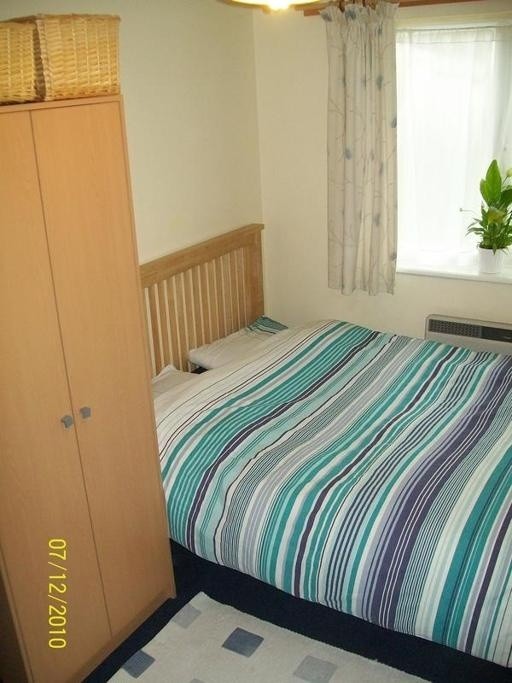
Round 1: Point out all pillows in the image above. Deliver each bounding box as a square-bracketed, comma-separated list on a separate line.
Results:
[151, 313, 290, 400]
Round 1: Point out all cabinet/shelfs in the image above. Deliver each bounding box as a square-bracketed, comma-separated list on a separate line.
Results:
[0, 93, 179, 683]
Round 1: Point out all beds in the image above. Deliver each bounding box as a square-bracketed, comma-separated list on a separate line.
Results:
[139, 222, 512, 672]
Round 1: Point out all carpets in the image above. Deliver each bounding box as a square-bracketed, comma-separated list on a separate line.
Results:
[109, 590, 433, 683]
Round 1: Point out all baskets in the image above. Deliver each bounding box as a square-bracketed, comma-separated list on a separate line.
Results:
[0, 15, 121, 103]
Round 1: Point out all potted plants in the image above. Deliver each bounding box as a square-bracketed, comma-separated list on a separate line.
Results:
[463, 159, 512, 274]
